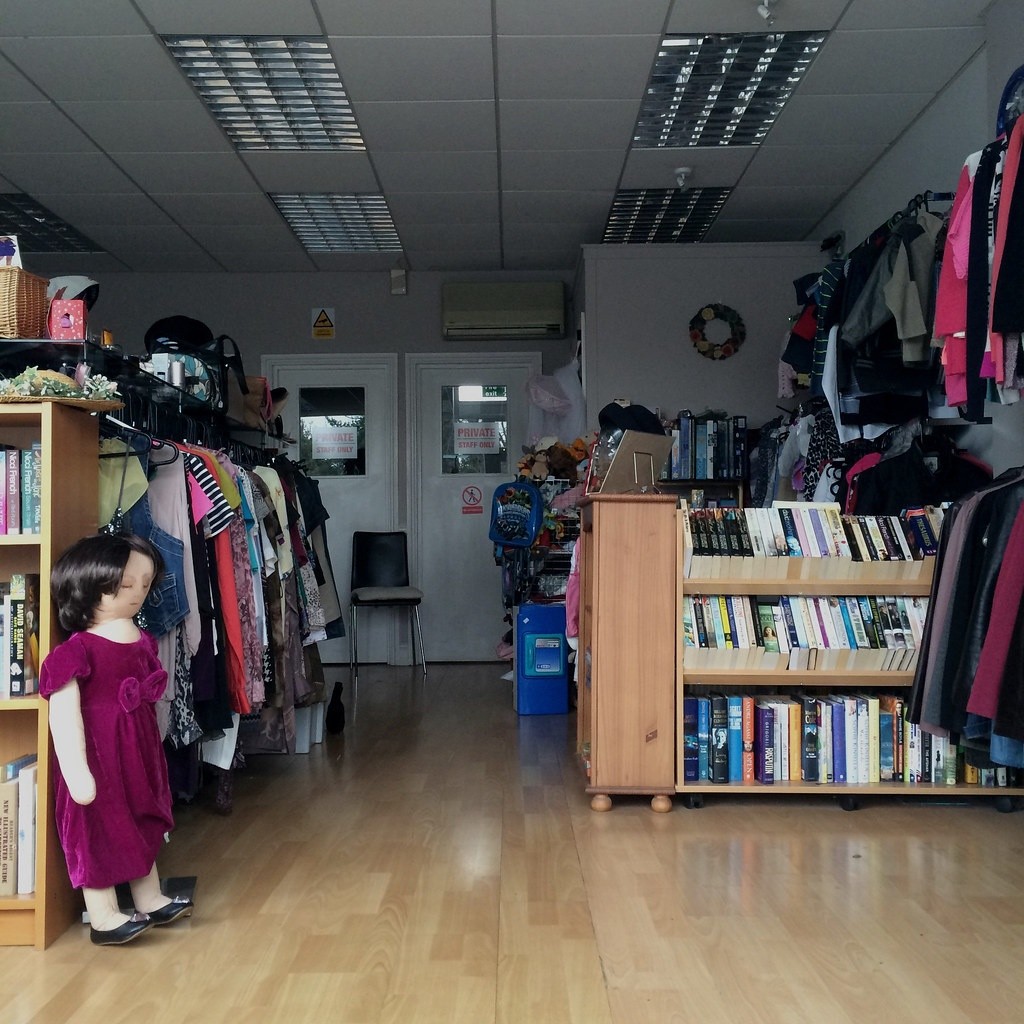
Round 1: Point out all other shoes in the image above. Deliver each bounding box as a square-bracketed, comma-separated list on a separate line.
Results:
[134, 896, 195, 925]
[90, 912, 154, 945]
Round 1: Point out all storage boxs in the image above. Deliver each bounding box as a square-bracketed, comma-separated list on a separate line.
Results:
[295, 702, 325, 754]
[45, 285, 88, 340]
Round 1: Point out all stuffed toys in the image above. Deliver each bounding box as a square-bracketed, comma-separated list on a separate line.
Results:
[515, 430, 598, 484]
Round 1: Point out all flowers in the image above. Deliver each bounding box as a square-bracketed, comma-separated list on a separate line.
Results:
[0, 363, 117, 399]
[688, 303, 748, 360]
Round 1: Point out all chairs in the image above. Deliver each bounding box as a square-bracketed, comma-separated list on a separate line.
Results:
[346, 529, 429, 679]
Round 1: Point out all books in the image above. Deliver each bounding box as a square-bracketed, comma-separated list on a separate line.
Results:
[680, 489, 953, 581]
[0, 574, 40, 700]
[683, 594, 930, 671]
[0, 752, 38, 898]
[659, 415, 748, 481]
[684, 694, 1024, 787]
[0, 438, 41, 535]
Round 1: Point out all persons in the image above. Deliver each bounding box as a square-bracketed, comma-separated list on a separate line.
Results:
[40, 533, 194, 947]
[762, 626, 777, 643]
[713, 729, 726, 749]
[878, 549, 889, 561]
[879, 606, 891, 631]
[786, 536, 802, 557]
[830, 597, 839, 608]
[896, 702, 903, 715]
[743, 741, 752, 752]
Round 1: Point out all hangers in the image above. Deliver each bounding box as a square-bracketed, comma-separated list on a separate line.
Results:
[98, 387, 268, 470]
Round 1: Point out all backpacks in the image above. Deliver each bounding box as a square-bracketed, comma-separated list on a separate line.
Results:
[489, 475, 543, 546]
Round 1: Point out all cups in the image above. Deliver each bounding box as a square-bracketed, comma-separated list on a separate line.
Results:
[170, 361, 185, 388]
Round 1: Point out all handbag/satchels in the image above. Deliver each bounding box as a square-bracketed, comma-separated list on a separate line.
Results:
[226, 374, 272, 432]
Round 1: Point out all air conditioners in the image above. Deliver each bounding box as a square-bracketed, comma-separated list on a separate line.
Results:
[440, 282, 566, 341]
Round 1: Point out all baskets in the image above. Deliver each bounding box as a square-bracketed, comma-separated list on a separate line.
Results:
[0, 265, 50, 340]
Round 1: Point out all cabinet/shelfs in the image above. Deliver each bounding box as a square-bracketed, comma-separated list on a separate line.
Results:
[576, 494, 677, 811]
[679, 507, 1023, 811]
[0, 402, 101, 951]
[656, 476, 746, 506]
[525, 474, 580, 603]
